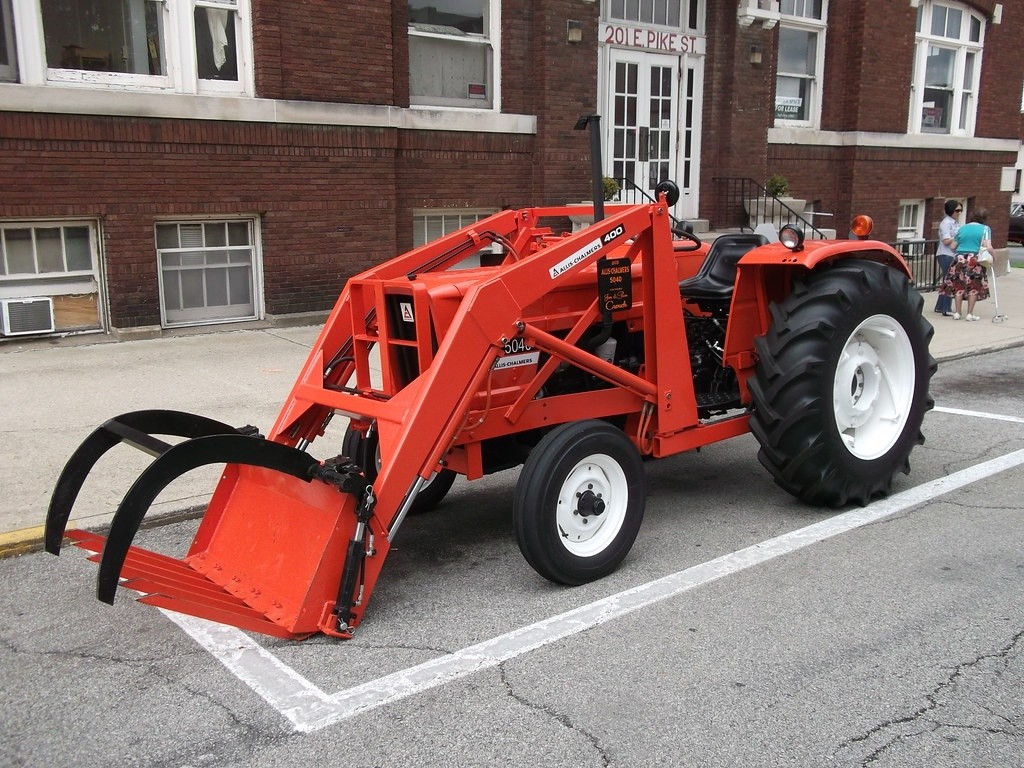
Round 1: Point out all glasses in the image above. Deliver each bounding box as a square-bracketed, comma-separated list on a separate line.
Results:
[955, 209, 963, 213]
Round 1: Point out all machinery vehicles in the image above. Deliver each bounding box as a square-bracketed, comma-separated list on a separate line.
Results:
[44, 114, 940, 643]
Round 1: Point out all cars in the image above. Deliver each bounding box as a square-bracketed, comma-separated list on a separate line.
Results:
[1008, 202, 1024, 247]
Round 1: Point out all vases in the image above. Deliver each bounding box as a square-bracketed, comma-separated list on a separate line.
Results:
[743, 173, 807, 231]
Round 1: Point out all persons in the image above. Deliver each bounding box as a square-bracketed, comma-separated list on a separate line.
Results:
[934, 200, 964, 317]
[937, 208, 996, 322]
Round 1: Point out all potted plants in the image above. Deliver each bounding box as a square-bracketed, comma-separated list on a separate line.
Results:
[565, 177, 624, 232]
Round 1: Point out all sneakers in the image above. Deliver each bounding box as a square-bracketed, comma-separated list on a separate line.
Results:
[966, 314, 980, 321]
[943, 311, 954, 316]
[953, 312, 963, 319]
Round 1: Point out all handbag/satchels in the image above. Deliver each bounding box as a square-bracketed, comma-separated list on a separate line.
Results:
[977, 246, 994, 267]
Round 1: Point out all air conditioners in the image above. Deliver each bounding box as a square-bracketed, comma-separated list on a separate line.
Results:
[899, 238, 926, 259]
[0, 297, 55, 336]
